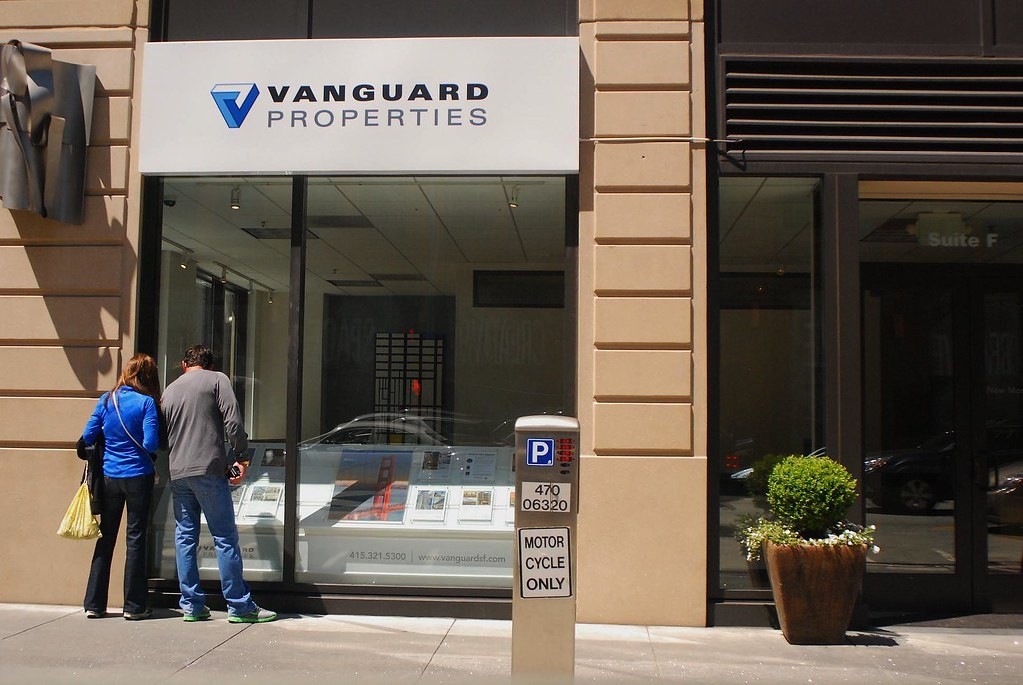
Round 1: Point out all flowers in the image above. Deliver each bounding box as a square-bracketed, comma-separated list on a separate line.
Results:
[739, 453, 881, 561]
[732, 456, 787, 556]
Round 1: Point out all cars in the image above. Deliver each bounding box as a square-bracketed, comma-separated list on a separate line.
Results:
[729, 441, 1023, 530]
[298, 404, 565, 446]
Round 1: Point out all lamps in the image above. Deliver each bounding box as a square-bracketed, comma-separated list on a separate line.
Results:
[248, 280, 253, 294]
[507, 186, 521, 208]
[220, 269, 228, 283]
[231, 188, 242, 210]
[267, 291, 272, 303]
[181, 253, 192, 270]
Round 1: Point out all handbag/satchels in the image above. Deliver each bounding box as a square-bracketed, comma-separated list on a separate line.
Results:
[56, 461, 103, 541]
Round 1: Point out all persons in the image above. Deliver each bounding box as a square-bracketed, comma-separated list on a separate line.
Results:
[159, 345, 277, 623]
[82, 353, 160, 621]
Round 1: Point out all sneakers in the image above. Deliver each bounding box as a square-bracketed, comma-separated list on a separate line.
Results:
[228, 605, 278, 623]
[183, 605, 211, 621]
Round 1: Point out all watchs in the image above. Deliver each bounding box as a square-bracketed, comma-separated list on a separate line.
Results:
[241, 461, 249, 467]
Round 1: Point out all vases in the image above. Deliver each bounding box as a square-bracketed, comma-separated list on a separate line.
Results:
[745, 552, 772, 591]
[761, 539, 867, 645]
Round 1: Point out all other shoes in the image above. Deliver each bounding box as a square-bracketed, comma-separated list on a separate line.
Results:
[123, 607, 153, 620]
[85, 609, 107, 618]
